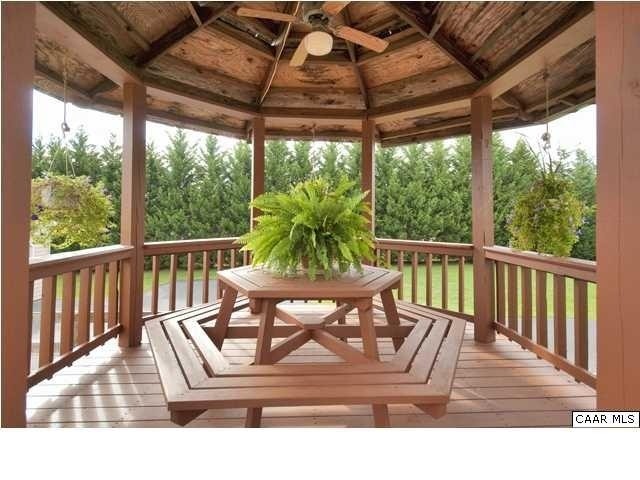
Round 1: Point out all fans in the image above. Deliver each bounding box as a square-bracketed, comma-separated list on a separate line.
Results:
[236, 1, 390, 67]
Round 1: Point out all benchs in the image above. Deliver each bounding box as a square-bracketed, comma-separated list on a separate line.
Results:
[143, 296, 467, 427]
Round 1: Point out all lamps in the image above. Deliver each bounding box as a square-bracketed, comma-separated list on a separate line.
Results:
[302, 1, 334, 56]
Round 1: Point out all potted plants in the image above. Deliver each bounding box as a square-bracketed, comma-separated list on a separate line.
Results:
[31, 174, 119, 251]
[233, 174, 382, 280]
[503, 157, 587, 259]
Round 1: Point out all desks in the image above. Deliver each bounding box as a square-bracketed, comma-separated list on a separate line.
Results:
[200, 258, 419, 428]
